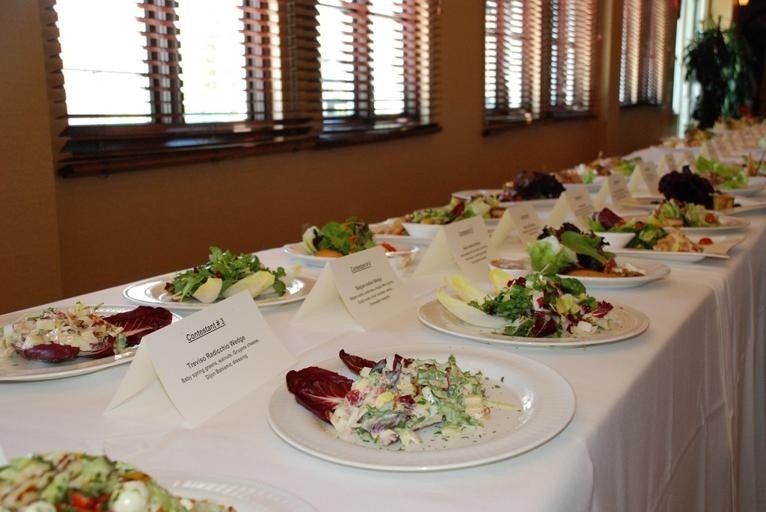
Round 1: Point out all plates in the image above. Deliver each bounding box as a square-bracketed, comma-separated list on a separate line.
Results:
[618, 214, 751, 232]
[711, 179, 763, 197]
[624, 195, 765, 216]
[489, 255, 669, 295]
[558, 176, 605, 194]
[151, 470, 315, 512]
[453, 187, 559, 208]
[0, 302, 184, 384]
[265, 341, 579, 474]
[416, 293, 651, 348]
[280, 234, 421, 267]
[540, 231, 748, 267]
[121, 271, 317, 311]
[368, 216, 479, 244]
